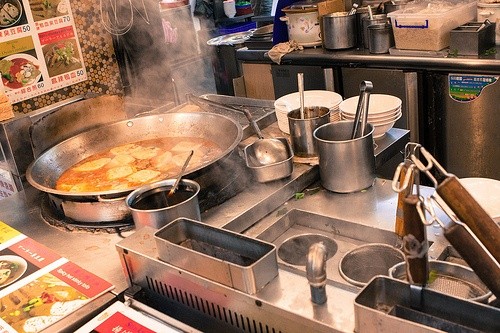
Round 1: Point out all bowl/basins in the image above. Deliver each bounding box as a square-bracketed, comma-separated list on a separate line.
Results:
[274, 90, 343, 134]
[0, 0, 22, 27]
[125, 179, 201, 233]
[289, 107, 330, 158]
[435, 177, 500, 222]
[313, 120, 374, 193]
[340, 95, 403, 138]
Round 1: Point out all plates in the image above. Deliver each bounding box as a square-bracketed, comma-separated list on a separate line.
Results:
[0, 54, 42, 89]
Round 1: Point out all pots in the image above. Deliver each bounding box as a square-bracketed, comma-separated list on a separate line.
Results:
[26, 112, 242, 203]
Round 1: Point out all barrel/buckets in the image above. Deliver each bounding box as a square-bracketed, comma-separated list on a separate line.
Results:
[321, 12, 360, 49]
[207, 32, 256, 97]
[363, 14, 391, 53]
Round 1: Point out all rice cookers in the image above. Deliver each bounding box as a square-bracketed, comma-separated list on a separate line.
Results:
[280, 4, 322, 49]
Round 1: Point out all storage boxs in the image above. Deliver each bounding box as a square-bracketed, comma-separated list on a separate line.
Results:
[386, 0, 479, 51]
